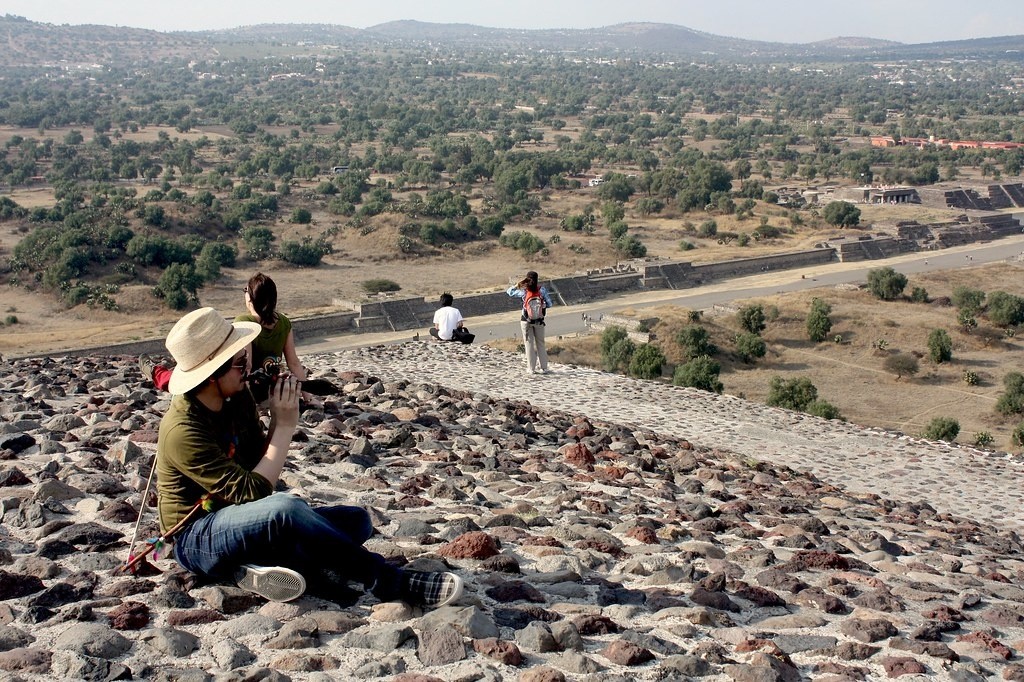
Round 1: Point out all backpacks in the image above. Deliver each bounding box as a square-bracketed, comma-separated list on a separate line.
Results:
[523, 285, 543, 320]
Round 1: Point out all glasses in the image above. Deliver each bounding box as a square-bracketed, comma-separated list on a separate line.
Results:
[219, 348, 247, 375]
[243, 287, 249, 294]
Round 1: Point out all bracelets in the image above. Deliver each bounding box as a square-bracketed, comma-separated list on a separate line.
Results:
[304, 398, 312, 403]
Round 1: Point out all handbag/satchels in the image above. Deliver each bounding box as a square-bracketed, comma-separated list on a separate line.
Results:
[452, 327, 475, 344]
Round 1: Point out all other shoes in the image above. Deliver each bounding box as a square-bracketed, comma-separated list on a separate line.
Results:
[138, 353, 158, 382]
[540, 368, 548, 374]
[527, 369, 535, 375]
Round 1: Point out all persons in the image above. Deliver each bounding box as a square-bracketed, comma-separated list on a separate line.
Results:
[507, 271, 552, 373]
[138, 272, 325, 409]
[429, 294, 463, 340]
[157, 307, 463, 609]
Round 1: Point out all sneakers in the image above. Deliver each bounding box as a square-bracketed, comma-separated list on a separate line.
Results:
[234, 564, 306, 603]
[381, 566, 463, 610]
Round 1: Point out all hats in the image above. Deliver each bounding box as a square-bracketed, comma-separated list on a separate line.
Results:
[527, 271, 538, 279]
[167, 307, 262, 395]
